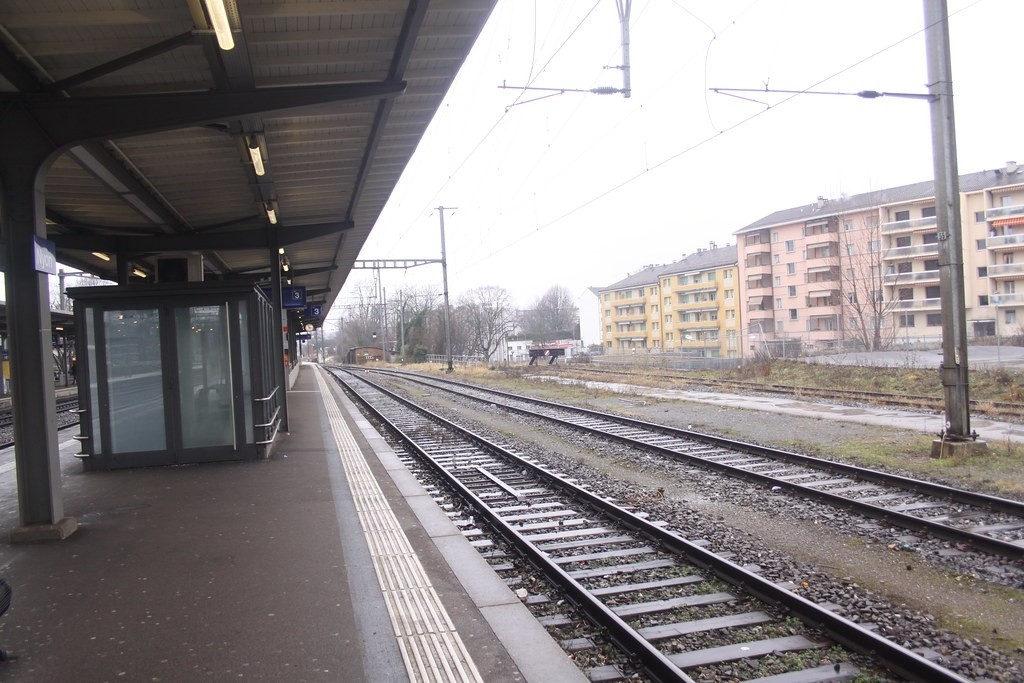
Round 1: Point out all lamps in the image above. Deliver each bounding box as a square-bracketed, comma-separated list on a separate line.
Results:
[265, 199, 278, 224]
[204, 0, 235, 51]
[248, 135, 265, 177]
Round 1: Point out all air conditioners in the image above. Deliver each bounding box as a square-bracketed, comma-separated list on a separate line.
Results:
[155, 255, 204, 282]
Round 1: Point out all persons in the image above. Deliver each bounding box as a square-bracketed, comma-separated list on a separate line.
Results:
[71, 358, 77, 385]
